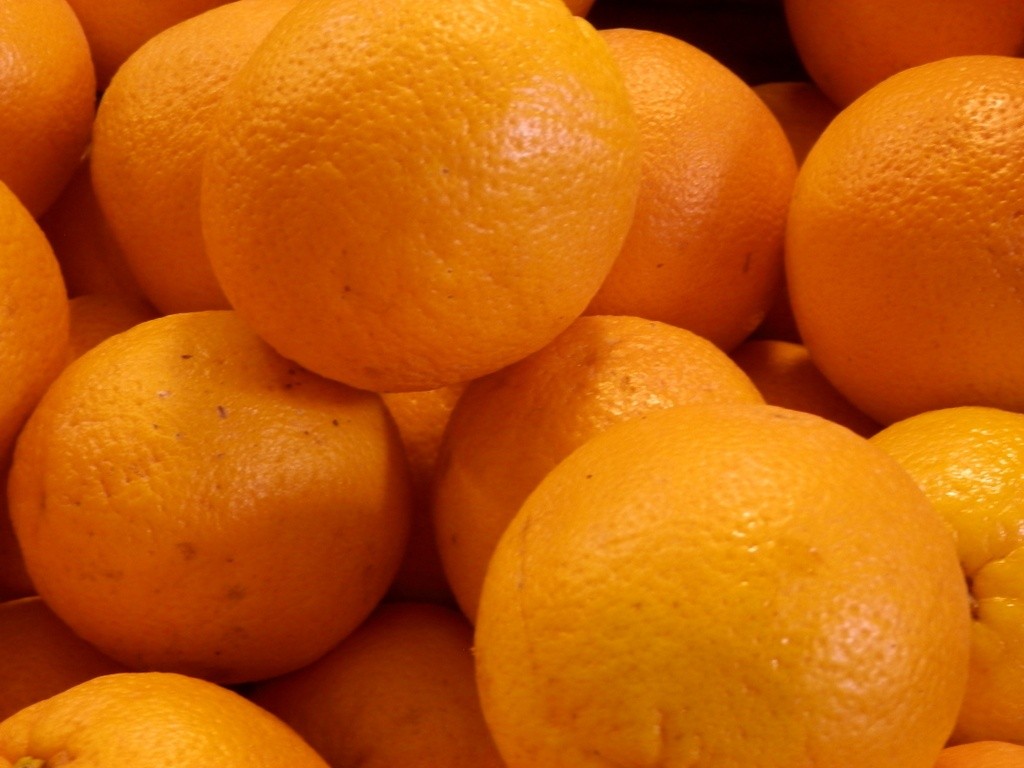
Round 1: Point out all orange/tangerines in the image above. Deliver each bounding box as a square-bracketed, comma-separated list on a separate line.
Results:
[0, 0, 1024, 768]
